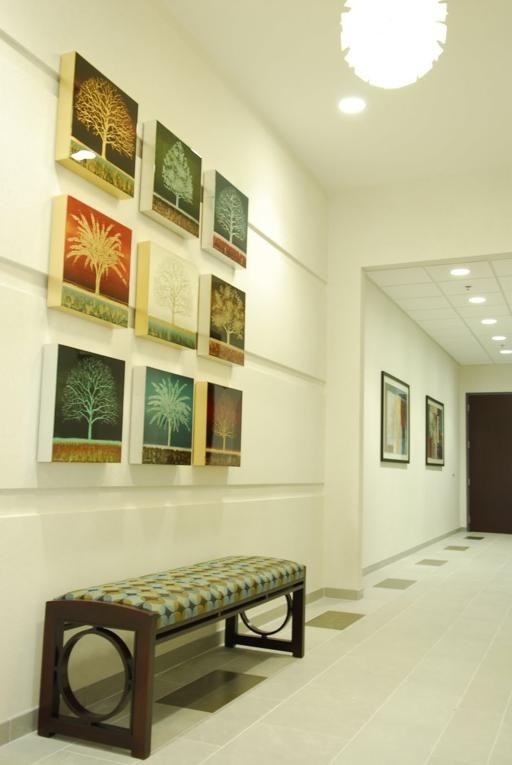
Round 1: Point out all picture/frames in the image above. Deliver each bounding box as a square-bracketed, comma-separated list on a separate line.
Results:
[380, 370, 410, 463]
[425, 395, 445, 466]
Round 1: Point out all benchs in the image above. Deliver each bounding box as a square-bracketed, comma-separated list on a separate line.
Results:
[36, 557, 308, 760]
